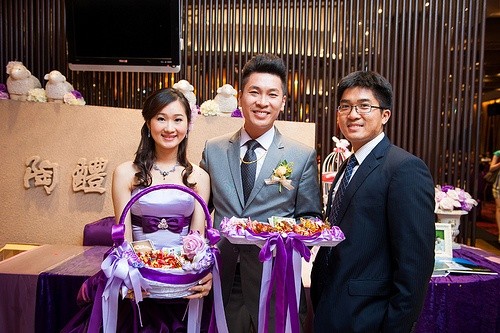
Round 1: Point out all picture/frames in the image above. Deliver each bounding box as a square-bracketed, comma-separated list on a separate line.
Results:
[435, 223, 452, 259]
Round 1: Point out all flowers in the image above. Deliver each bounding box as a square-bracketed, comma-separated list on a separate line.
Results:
[200, 99, 219, 116]
[265, 161, 295, 194]
[64, 90, 86, 106]
[180, 232, 206, 260]
[433, 184, 478, 212]
[27, 87, 47, 102]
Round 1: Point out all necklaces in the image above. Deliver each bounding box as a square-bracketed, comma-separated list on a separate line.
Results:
[153, 162, 179, 180]
[240, 152, 266, 164]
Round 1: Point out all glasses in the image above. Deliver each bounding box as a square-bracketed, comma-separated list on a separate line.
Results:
[338, 103, 387, 114]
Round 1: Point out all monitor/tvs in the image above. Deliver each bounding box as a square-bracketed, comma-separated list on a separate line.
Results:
[64, 0, 182, 73]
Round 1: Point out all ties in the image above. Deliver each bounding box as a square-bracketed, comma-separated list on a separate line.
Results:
[241, 140, 261, 206]
[328, 153, 359, 227]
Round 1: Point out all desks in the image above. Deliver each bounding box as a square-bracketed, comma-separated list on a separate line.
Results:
[0, 244, 500, 333]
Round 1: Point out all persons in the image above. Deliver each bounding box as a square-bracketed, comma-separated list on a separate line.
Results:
[64, 88, 212, 333]
[491, 151, 500, 242]
[200, 54, 322, 333]
[310, 71, 435, 333]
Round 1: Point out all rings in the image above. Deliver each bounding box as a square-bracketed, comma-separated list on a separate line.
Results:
[128, 290, 131, 293]
[129, 294, 133, 299]
[200, 293, 202, 299]
[201, 285, 204, 292]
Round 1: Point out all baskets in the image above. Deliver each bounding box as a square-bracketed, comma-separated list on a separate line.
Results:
[119, 184, 213, 299]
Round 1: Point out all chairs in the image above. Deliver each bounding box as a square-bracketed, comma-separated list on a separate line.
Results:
[83, 216, 116, 247]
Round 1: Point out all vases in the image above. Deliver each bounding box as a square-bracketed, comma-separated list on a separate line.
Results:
[436, 210, 468, 241]
